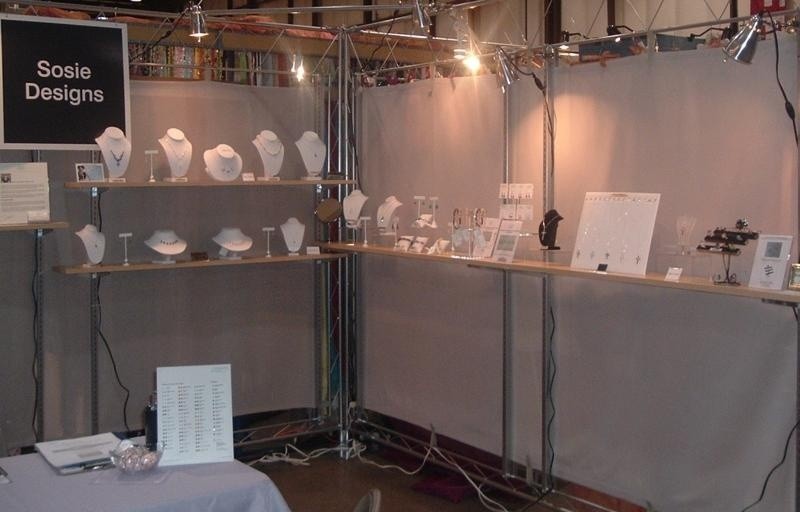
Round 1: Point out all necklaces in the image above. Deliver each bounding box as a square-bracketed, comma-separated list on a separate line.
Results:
[380, 199, 398, 223]
[575, 193, 657, 265]
[87, 134, 323, 250]
[541, 213, 561, 241]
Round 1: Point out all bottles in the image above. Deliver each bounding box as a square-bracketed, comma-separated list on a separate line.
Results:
[142, 391, 157, 451]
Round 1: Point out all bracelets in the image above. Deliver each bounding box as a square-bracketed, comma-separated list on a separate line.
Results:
[453, 208, 460, 230]
[397, 237, 411, 242]
[473, 207, 484, 228]
[411, 239, 424, 245]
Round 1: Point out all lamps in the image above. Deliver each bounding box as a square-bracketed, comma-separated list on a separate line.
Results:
[556, 24, 635, 51]
[719, 6, 799, 147]
[409, 0, 532, 95]
[187, 2, 209, 44]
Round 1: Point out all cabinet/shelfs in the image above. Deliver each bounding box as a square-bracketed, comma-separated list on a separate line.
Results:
[52, 179, 356, 462]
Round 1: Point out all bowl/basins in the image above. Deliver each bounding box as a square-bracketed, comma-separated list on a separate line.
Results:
[109, 446, 163, 474]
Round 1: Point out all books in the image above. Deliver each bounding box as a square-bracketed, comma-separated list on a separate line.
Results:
[33, 429, 133, 475]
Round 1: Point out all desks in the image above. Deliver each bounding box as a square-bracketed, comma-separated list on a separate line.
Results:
[0, 436, 272, 510]
